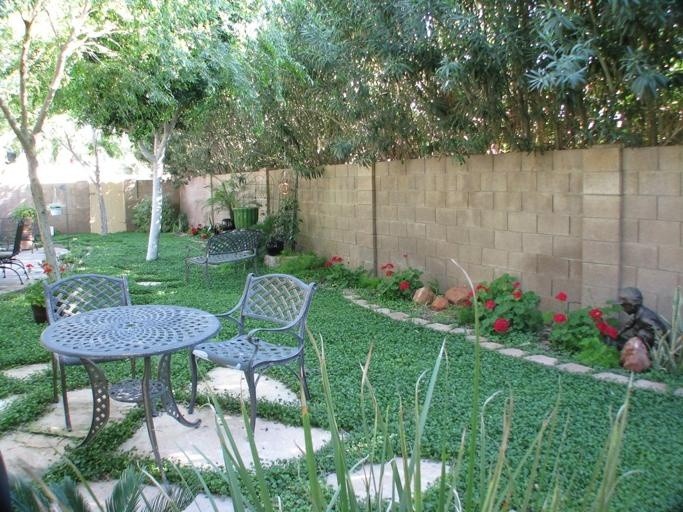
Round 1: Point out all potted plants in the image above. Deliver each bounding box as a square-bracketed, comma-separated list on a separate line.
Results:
[9, 203, 37, 250]
[253, 214, 284, 256]
[48, 202, 65, 216]
[26, 280, 45, 323]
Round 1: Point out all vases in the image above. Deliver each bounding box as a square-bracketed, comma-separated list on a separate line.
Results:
[222, 218, 233, 231]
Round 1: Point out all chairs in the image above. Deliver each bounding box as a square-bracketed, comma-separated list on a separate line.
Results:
[0, 219, 29, 285]
[43, 273, 159, 431]
[190, 272, 319, 439]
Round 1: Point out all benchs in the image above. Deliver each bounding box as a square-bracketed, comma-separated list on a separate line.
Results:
[186, 231, 260, 288]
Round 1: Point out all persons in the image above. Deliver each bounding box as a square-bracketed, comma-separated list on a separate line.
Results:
[612, 286, 670, 357]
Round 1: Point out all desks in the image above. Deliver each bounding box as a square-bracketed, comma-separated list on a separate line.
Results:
[39, 303, 221, 496]
